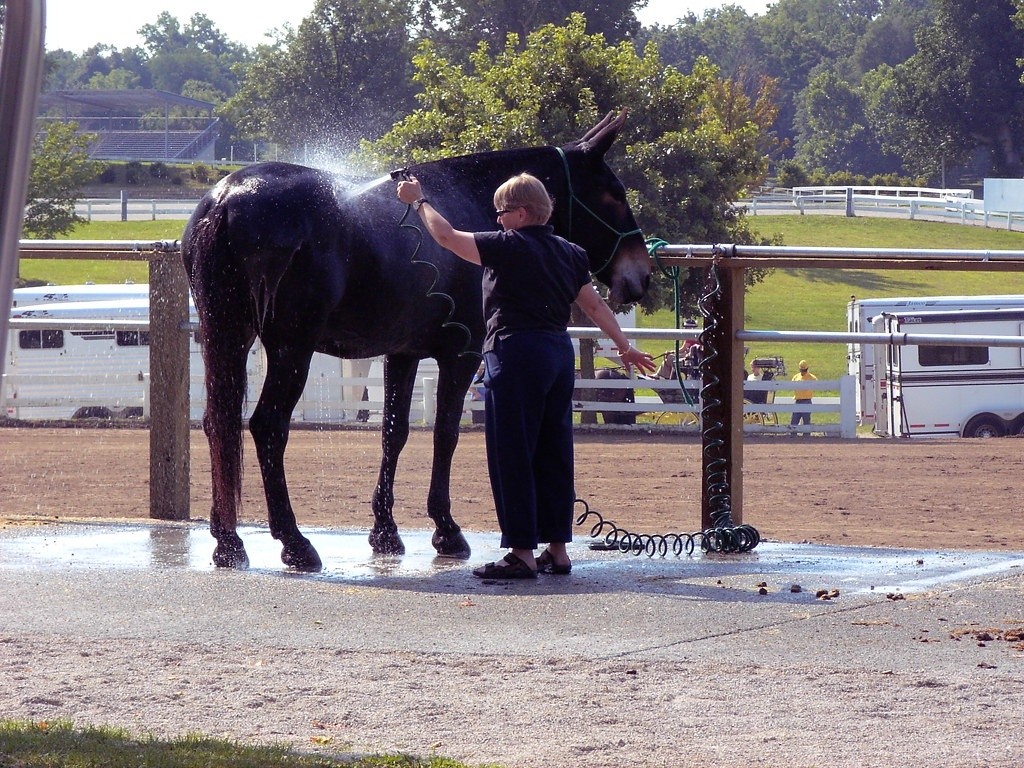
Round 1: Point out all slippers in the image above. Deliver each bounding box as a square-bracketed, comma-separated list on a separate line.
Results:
[473, 553, 538, 579]
[534, 549, 572, 574]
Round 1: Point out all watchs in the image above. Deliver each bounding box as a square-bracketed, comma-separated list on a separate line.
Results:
[413, 198, 429, 212]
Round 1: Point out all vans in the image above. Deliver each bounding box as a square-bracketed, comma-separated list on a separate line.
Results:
[0, 281, 343, 421]
[848, 294, 1024, 434]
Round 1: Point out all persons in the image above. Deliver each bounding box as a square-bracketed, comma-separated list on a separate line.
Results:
[646, 319, 703, 380]
[791, 359, 817, 436]
[747, 359, 763, 381]
[397, 172, 657, 579]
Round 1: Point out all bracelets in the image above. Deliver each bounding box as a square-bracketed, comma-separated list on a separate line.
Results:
[617, 343, 632, 357]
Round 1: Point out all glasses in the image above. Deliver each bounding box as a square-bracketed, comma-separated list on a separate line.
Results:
[496, 208, 516, 217]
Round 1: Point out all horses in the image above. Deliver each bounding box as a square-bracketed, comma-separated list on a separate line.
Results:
[182, 105, 652, 569]
